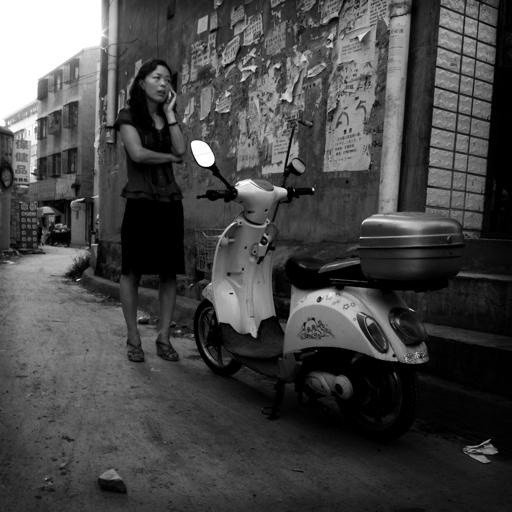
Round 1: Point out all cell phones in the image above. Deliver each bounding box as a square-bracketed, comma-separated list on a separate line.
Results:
[165, 90, 172, 103]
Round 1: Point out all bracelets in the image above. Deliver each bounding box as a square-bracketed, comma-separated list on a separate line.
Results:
[168, 122, 177, 127]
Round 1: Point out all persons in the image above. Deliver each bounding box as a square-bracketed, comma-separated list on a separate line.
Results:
[114, 58, 188, 362]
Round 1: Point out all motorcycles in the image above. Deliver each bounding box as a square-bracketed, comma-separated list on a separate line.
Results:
[189, 138, 465, 443]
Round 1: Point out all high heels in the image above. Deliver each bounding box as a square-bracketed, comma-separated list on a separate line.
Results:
[156, 342, 178, 361]
[127, 340, 144, 362]
